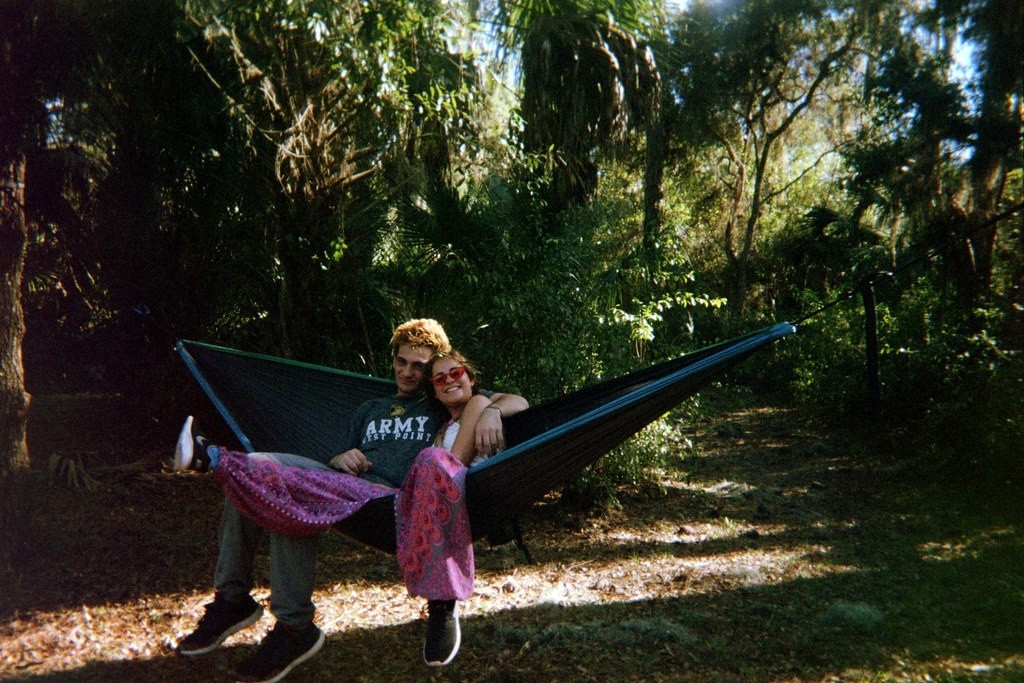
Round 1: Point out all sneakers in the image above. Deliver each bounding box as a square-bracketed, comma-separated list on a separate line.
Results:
[179, 592, 263, 655]
[422, 599, 462, 666]
[175, 415, 212, 474]
[235, 620, 325, 683]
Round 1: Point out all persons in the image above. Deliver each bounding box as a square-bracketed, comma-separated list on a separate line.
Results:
[180, 319, 528, 683]
[175, 351, 504, 665]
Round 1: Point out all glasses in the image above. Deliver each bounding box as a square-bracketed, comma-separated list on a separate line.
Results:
[430, 366, 465, 385]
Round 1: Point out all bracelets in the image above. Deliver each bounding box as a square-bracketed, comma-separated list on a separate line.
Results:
[487, 406, 502, 416]
